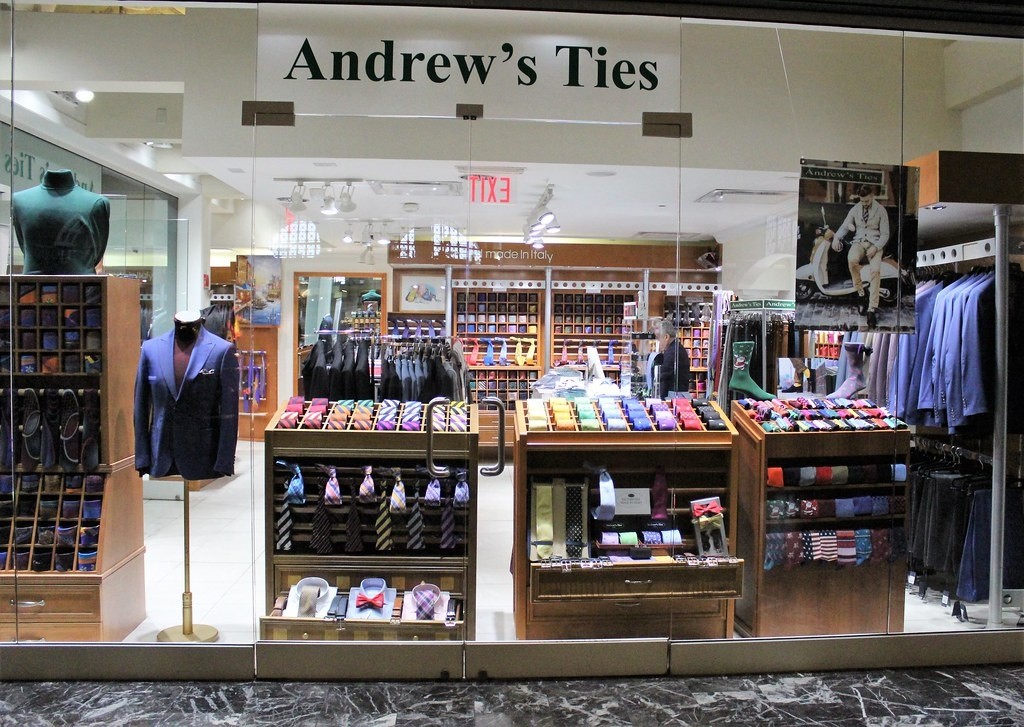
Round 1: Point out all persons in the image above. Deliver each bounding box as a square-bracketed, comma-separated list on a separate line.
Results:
[12, 169, 110, 275]
[368, 304, 376, 319]
[655, 321, 691, 402]
[832, 184, 889, 328]
[362, 290, 381, 310]
[133, 311, 239, 479]
[318, 314, 333, 346]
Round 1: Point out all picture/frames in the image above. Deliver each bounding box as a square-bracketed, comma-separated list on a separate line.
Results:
[398, 275, 447, 312]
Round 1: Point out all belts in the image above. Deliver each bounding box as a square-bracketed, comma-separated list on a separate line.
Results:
[727, 313, 797, 400]
[271, 596, 288, 617]
[707, 289, 739, 393]
[541, 556, 614, 574]
[444, 599, 457, 629]
[672, 552, 740, 567]
[390, 598, 403, 625]
[324, 596, 349, 631]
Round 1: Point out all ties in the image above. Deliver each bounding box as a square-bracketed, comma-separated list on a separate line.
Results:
[274, 460, 470, 554]
[238, 349, 243, 391]
[526, 397, 727, 431]
[251, 366, 261, 412]
[260, 350, 266, 398]
[665, 300, 712, 327]
[389, 318, 446, 340]
[0, 388, 100, 473]
[416, 588, 436, 620]
[689, 372, 706, 398]
[601, 531, 639, 545]
[277, 396, 468, 432]
[453, 337, 536, 366]
[677, 329, 708, 368]
[590, 464, 616, 521]
[641, 529, 682, 544]
[244, 365, 248, 411]
[458, 292, 537, 332]
[0, 474, 105, 572]
[248, 350, 254, 395]
[555, 295, 634, 334]
[553, 339, 619, 368]
[536, 485, 554, 561]
[608, 372, 616, 381]
[469, 370, 538, 410]
[566, 485, 582, 559]
[0, 285, 101, 373]
[651, 464, 668, 520]
[297, 585, 321, 618]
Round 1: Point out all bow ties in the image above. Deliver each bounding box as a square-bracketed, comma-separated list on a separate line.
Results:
[355, 591, 384, 608]
[692, 499, 724, 517]
[691, 513, 724, 530]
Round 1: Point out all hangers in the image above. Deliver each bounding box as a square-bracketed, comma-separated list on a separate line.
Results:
[346, 334, 460, 364]
[724, 311, 800, 328]
[910, 428, 1024, 488]
[914, 255, 993, 286]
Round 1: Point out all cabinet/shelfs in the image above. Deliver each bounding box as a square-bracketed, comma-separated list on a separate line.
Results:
[541, 292, 635, 385]
[454, 291, 541, 408]
[662, 298, 715, 401]
[511, 401, 745, 639]
[0, 274, 149, 643]
[265, 398, 479, 641]
[350, 311, 380, 335]
[731, 400, 909, 637]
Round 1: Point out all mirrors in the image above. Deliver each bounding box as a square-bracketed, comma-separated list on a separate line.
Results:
[294, 271, 387, 399]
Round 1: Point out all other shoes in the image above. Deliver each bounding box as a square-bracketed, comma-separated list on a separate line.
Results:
[857, 290, 869, 316]
[868, 311, 878, 328]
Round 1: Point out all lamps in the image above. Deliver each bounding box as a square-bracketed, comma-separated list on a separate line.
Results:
[310, 184, 329, 205]
[284, 184, 311, 212]
[538, 205, 554, 223]
[546, 217, 562, 233]
[322, 185, 338, 216]
[74, 87, 93, 104]
[524, 223, 540, 236]
[524, 231, 535, 245]
[529, 214, 545, 231]
[533, 239, 545, 250]
[341, 219, 394, 245]
[335, 186, 357, 214]
